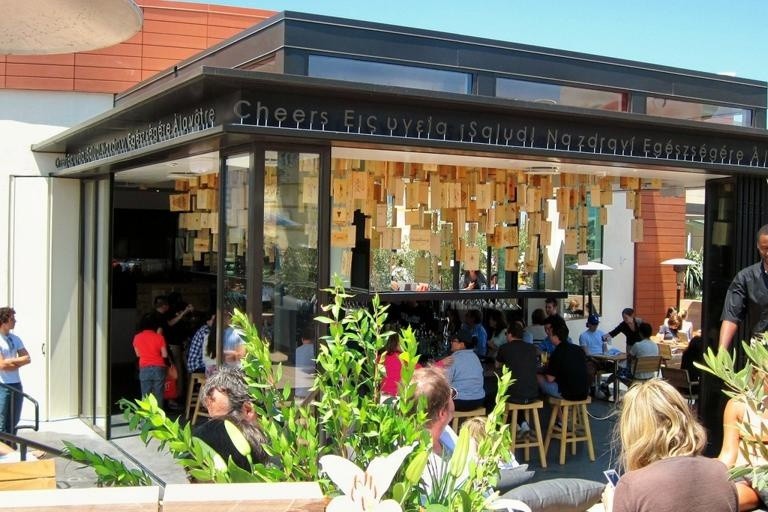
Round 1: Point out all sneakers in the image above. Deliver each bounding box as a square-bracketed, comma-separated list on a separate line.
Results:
[600, 383, 612, 396]
[518, 422, 530, 439]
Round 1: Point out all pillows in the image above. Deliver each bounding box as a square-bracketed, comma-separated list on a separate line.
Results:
[743, 464, 768, 509]
[489, 468, 536, 490]
[494, 477, 606, 512]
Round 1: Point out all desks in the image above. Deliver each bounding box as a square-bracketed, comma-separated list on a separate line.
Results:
[0, 458, 57, 491]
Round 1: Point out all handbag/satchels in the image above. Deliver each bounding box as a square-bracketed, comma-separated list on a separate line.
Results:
[164, 360, 178, 379]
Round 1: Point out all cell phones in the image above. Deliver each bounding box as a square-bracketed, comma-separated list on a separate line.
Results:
[603, 469, 620, 489]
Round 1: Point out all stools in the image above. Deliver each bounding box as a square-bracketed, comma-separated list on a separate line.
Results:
[192, 377, 212, 426]
[452, 407, 486, 436]
[545, 396, 595, 465]
[502, 400, 549, 469]
[186, 373, 205, 420]
[262, 312, 274, 342]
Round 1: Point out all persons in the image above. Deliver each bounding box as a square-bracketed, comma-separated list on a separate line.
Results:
[0, 306, 32, 451]
[717, 224, 768, 355]
[133, 270, 693, 481]
[714, 360, 768, 512]
[600, 377, 740, 512]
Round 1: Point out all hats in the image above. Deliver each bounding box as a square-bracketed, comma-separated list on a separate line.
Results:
[451, 330, 473, 344]
[588, 314, 600, 324]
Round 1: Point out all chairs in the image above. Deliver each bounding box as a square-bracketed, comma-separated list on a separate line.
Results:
[620, 330, 700, 410]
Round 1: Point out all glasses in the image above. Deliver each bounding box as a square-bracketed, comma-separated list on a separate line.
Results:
[6, 336, 14, 350]
